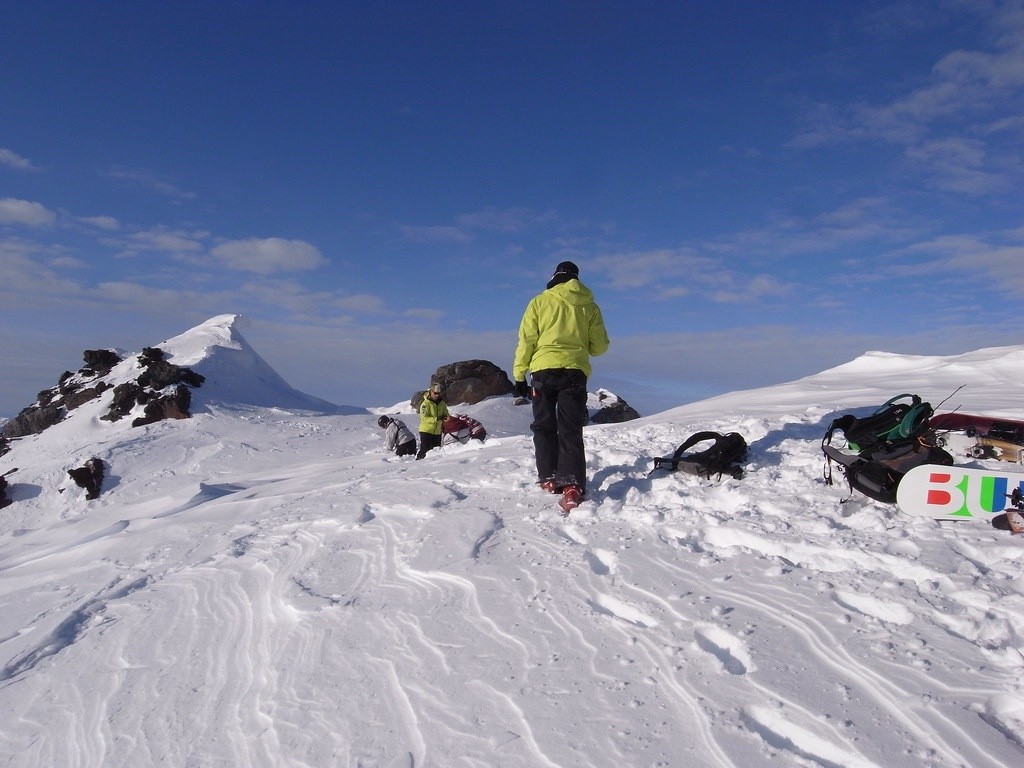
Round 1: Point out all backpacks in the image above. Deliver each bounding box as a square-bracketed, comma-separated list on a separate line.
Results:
[821, 393, 934, 466]
[847, 437, 954, 503]
[655, 431, 749, 480]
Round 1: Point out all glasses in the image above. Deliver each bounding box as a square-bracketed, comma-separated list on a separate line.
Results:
[434, 392, 441, 395]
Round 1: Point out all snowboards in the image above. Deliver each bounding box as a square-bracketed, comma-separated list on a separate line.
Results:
[928, 413, 1024, 448]
[895, 462, 1024, 524]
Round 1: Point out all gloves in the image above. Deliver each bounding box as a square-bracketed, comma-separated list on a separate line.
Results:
[438, 415, 443, 421]
[515, 380, 528, 398]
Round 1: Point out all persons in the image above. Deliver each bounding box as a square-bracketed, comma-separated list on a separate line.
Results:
[416, 384, 450, 461]
[378, 415, 417, 456]
[440, 413, 486, 445]
[513, 260, 609, 510]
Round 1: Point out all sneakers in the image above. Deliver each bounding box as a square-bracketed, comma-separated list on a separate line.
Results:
[559, 485, 583, 512]
[541, 480, 558, 494]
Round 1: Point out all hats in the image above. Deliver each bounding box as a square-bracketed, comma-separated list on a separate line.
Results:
[431, 384, 441, 393]
[554, 262, 580, 277]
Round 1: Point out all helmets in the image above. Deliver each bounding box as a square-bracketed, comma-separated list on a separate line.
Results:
[378, 415, 389, 426]
